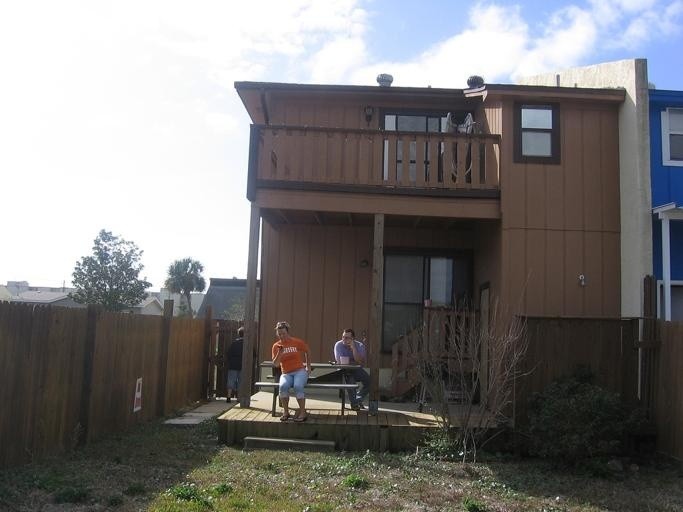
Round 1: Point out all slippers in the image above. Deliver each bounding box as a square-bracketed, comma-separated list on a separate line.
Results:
[280, 414, 290, 421]
[295, 415, 307, 422]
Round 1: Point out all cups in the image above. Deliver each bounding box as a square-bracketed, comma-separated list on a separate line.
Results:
[425, 299, 433, 307]
[339, 355, 349, 364]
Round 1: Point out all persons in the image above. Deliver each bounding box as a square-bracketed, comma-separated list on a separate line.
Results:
[333, 328, 369, 411]
[224, 326, 256, 404]
[271, 320, 311, 421]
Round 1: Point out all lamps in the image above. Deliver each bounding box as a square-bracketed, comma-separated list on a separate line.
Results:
[364, 104, 374, 126]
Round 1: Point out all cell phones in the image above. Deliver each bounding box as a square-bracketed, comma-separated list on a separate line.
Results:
[277, 345, 283, 349]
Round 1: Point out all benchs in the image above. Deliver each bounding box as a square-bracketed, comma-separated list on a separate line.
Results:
[266, 374, 360, 407]
[254, 382, 361, 416]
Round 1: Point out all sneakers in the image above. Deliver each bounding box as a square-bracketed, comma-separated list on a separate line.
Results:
[351, 400, 364, 410]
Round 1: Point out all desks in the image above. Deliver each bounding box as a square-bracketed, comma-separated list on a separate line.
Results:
[260, 361, 361, 416]
